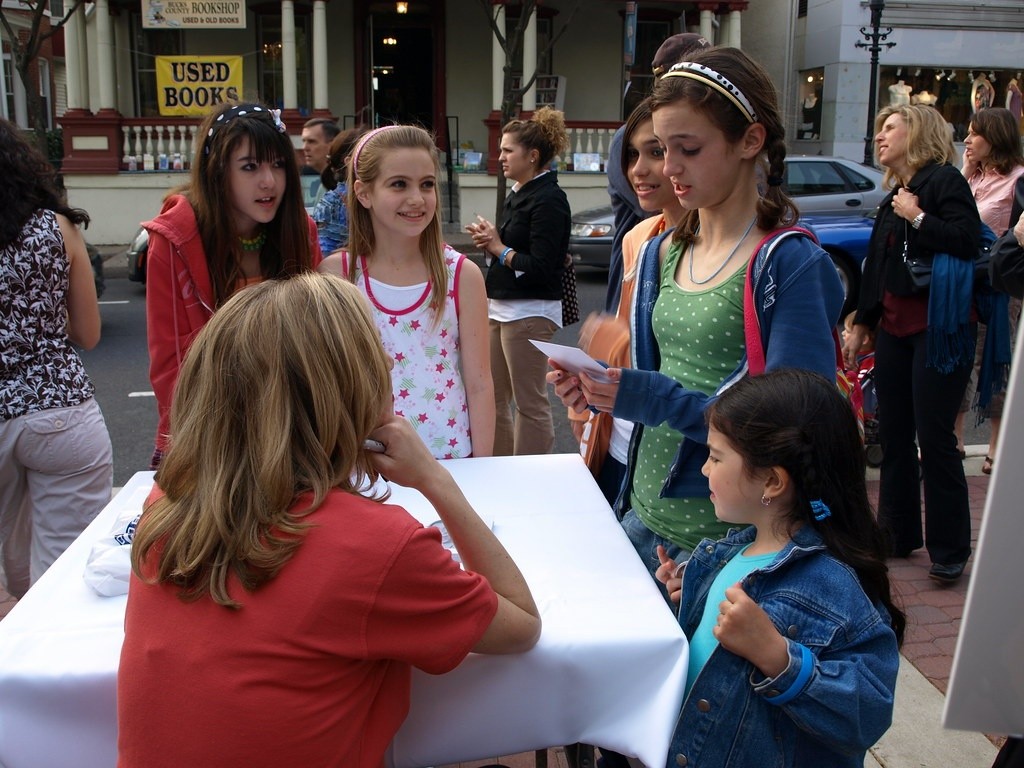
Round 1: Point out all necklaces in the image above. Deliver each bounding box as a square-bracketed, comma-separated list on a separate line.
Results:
[234, 232, 266, 252]
[691, 214, 760, 281]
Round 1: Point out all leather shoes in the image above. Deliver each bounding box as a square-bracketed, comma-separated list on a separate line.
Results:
[883, 550, 913, 558]
[928, 557, 968, 582]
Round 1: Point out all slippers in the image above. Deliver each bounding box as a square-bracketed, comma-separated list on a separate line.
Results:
[958, 450, 965, 459]
[982, 456, 993, 474]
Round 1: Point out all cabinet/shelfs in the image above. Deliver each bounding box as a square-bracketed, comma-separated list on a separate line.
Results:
[510, 74, 567, 111]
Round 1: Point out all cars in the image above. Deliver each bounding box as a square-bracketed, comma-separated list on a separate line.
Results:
[568, 153, 895, 270]
[792, 212, 876, 324]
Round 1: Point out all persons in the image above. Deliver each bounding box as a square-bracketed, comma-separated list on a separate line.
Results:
[296, 115, 370, 255]
[140, 97, 322, 477]
[801, 92, 821, 140]
[1004, 78, 1024, 126]
[115, 265, 543, 768]
[971, 72, 995, 114]
[546, 45, 849, 587]
[911, 89, 938, 108]
[0, 114, 113, 605]
[655, 363, 908, 768]
[604, 32, 713, 318]
[464, 103, 572, 459]
[312, 123, 498, 463]
[951, 106, 1024, 476]
[888, 80, 913, 107]
[835, 100, 988, 588]
[567, 91, 690, 507]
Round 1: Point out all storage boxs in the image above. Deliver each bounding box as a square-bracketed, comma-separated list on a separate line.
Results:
[453, 149, 475, 166]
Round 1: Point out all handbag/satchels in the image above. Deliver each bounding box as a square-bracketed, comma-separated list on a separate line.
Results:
[554, 253, 580, 326]
[903, 181, 990, 294]
[746, 223, 865, 451]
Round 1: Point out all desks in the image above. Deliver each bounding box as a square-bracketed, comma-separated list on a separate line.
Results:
[0, 454, 689, 768]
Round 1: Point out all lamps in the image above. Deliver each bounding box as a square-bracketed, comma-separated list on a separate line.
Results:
[897, 66, 1022, 84]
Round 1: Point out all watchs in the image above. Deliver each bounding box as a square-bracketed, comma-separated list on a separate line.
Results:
[910, 212, 926, 228]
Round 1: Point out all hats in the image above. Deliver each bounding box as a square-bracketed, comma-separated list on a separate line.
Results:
[653, 33, 711, 74]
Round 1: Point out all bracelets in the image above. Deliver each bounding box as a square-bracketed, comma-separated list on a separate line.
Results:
[500, 248, 513, 265]
